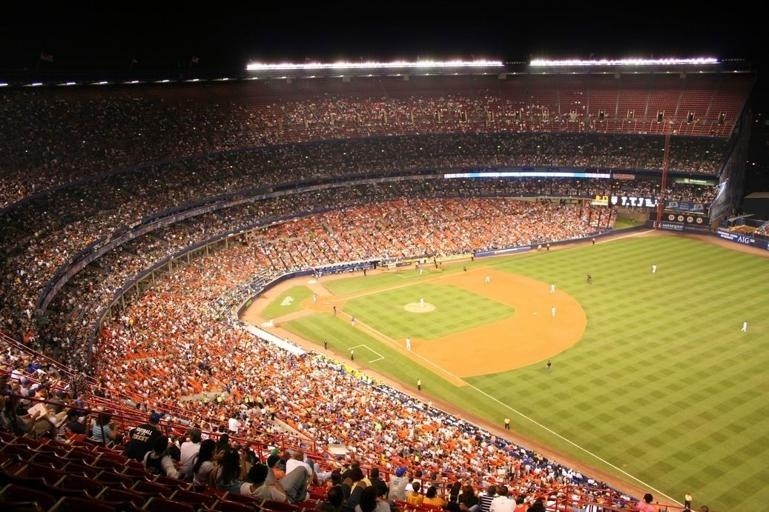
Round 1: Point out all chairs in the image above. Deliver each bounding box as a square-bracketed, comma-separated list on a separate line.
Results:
[1, 418, 314, 512]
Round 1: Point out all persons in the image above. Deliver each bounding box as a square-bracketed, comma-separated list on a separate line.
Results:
[0, 75, 238, 512]
[740, 321, 748, 333]
[238, 296, 691, 512]
[238, 85, 768, 294]
